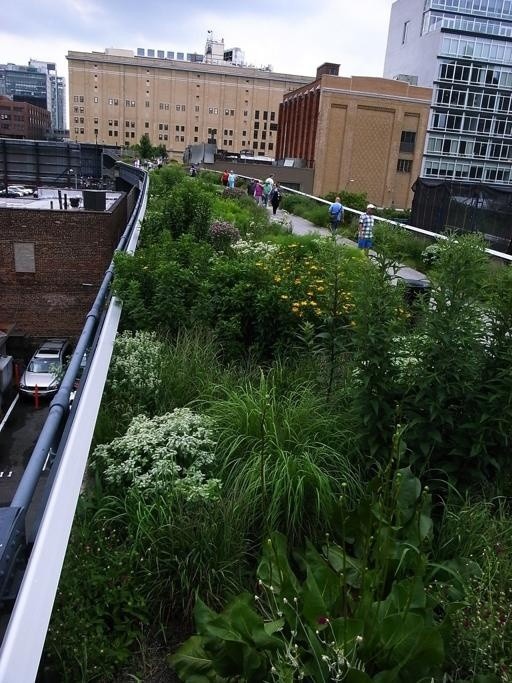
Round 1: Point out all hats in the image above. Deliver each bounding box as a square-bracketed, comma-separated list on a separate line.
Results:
[366, 203, 377, 210]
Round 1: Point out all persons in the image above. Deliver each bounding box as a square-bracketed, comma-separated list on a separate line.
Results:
[189, 164, 197, 176]
[328, 196, 344, 241]
[194, 162, 200, 173]
[247, 173, 283, 215]
[221, 168, 229, 185]
[229, 169, 235, 187]
[357, 203, 376, 258]
[133, 154, 168, 170]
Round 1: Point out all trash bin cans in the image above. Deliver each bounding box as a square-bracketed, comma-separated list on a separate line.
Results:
[82, 190, 105, 211]
[397, 279, 432, 334]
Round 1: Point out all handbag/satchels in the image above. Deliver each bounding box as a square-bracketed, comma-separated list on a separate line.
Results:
[329, 212, 339, 222]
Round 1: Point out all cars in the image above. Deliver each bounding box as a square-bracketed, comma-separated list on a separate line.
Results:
[0, 185, 33, 197]
[18, 338, 87, 410]
